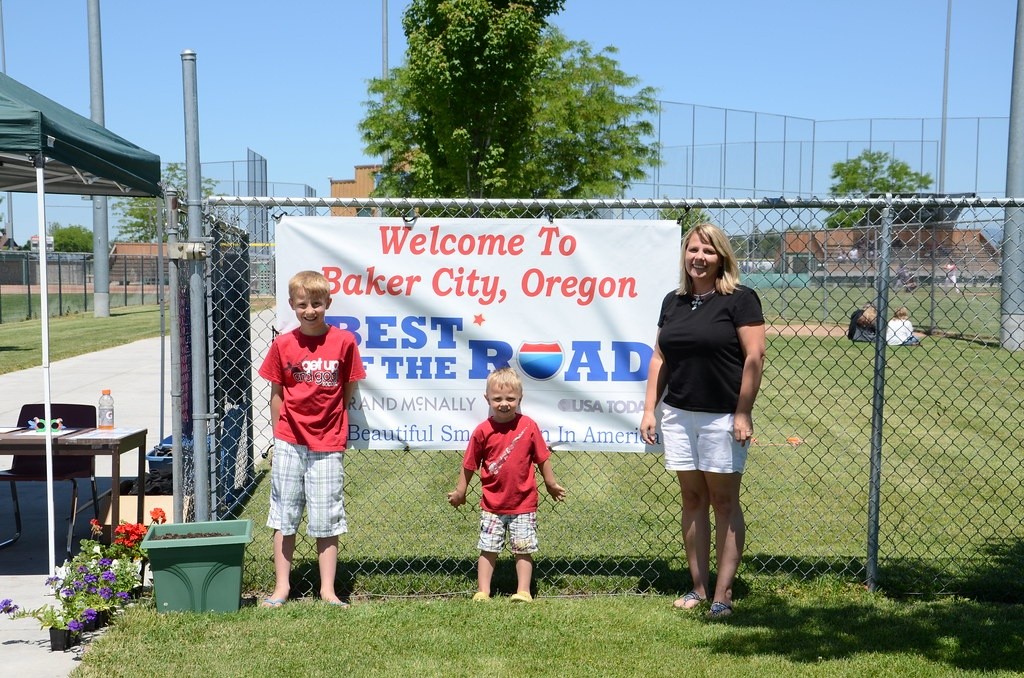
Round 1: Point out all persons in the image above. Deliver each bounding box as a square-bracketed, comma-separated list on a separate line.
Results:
[847, 301, 872, 340]
[446, 367, 567, 606]
[853, 305, 878, 343]
[256, 270, 367, 610]
[639, 223, 768, 622]
[892, 254, 964, 294]
[886, 307, 914, 347]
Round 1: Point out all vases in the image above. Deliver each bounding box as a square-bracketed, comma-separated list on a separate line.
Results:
[48, 583, 144, 650]
[138, 516, 255, 614]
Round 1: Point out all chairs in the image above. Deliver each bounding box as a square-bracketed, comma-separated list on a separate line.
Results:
[0, 404, 102, 559]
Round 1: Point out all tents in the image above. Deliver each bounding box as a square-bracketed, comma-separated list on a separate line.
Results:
[0, 71, 187, 605]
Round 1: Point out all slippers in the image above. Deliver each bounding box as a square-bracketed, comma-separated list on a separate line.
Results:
[473, 591, 492, 604]
[708, 601, 734, 620]
[671, 592, 707, 610]
[330, 601, 350, 610]
[263, 599, 287, 607]
[510, 591, 533, 603]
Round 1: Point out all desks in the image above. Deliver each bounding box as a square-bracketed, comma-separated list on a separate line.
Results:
[0, 428, 148, 547]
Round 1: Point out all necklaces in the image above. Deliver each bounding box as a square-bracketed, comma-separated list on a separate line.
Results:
[689, 288, 717, 310]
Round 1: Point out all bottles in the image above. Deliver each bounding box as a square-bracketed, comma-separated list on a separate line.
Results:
[97, 389, 114, 431]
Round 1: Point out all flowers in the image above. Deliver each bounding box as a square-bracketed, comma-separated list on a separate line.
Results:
[0, 507, 167, 635]
